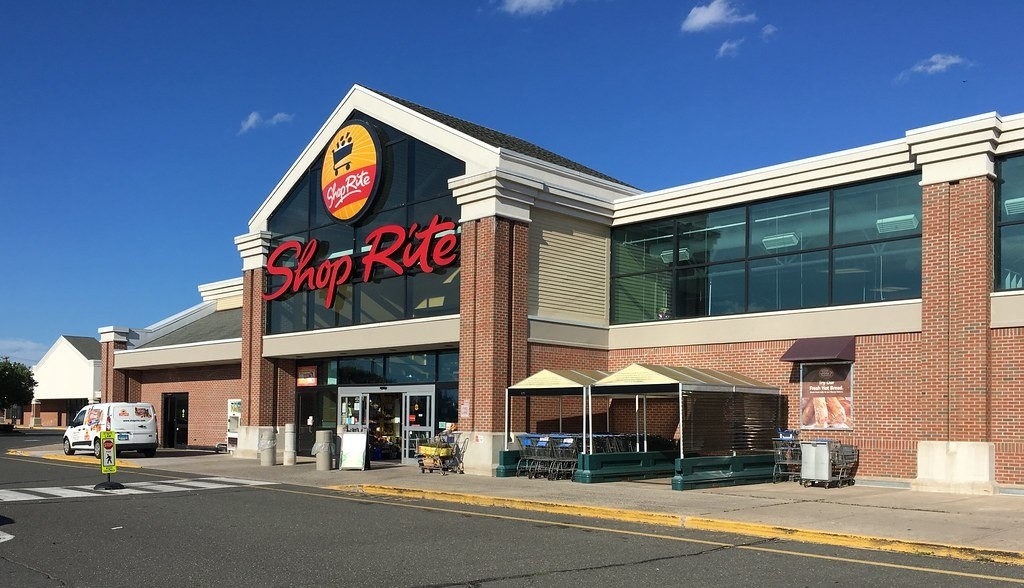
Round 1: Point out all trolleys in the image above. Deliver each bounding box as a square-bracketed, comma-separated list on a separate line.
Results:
[414, 431, 465, 476]
[770, 426, 861, 489]
[513, 429, 677, 481]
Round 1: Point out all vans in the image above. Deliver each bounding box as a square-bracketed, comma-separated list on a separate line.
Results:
[60, 401, 161, 458]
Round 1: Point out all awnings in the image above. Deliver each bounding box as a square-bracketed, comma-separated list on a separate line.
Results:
[779, 336, 855, 362]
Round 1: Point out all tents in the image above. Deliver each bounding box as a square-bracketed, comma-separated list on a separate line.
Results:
[496, 363, 782, 490]
